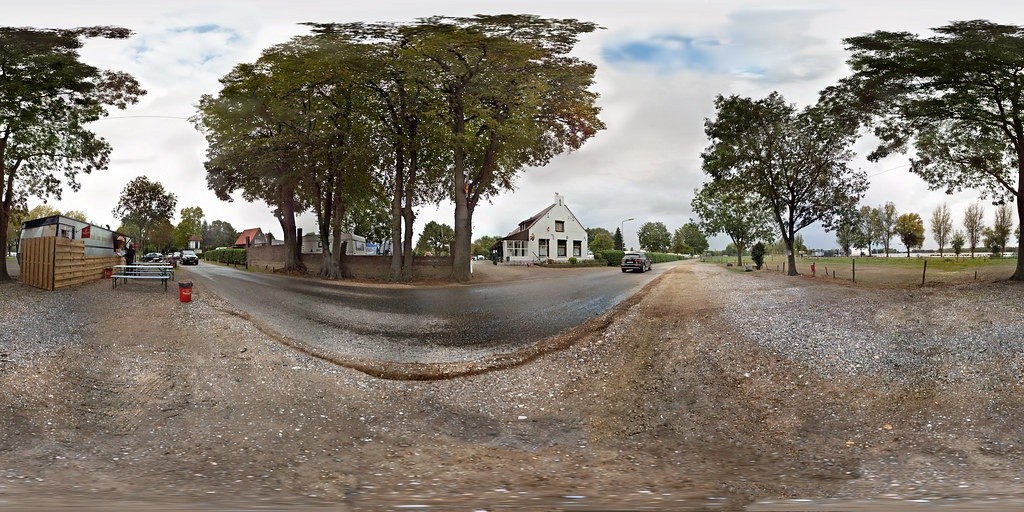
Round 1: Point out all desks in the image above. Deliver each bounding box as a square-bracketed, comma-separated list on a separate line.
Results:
[113, 264, 173, 275]
[131, 262, 170, 271]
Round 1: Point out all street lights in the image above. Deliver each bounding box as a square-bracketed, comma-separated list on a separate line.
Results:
[621, 217, 634, 252]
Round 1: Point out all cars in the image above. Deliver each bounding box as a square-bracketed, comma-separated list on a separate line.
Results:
[141, 252, 163, 262]
[179, 250, 198, 265]
[164, 252, 181, 260]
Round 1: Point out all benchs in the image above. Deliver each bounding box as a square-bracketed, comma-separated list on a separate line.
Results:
[110, 270, 174, 291]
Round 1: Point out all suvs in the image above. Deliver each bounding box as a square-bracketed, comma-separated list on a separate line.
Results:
[622, 253, 653, 273]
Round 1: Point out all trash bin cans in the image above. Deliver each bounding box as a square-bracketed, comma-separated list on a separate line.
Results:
[104, 267, 113, 278]
[178, 282, 193, 302]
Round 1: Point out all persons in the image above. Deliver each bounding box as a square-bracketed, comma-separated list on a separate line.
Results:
[123, 245, 135, 275]
